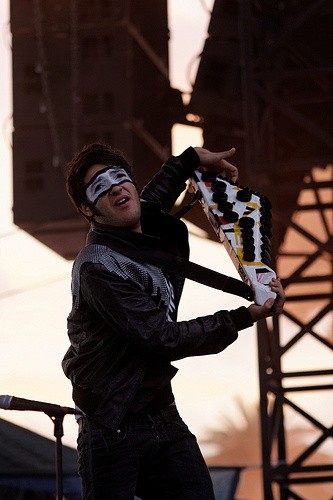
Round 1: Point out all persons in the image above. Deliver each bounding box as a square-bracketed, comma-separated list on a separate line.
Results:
[60, 140, 286, 499]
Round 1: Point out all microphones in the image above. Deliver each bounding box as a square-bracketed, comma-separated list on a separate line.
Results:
[0, 395, 82, 415]
